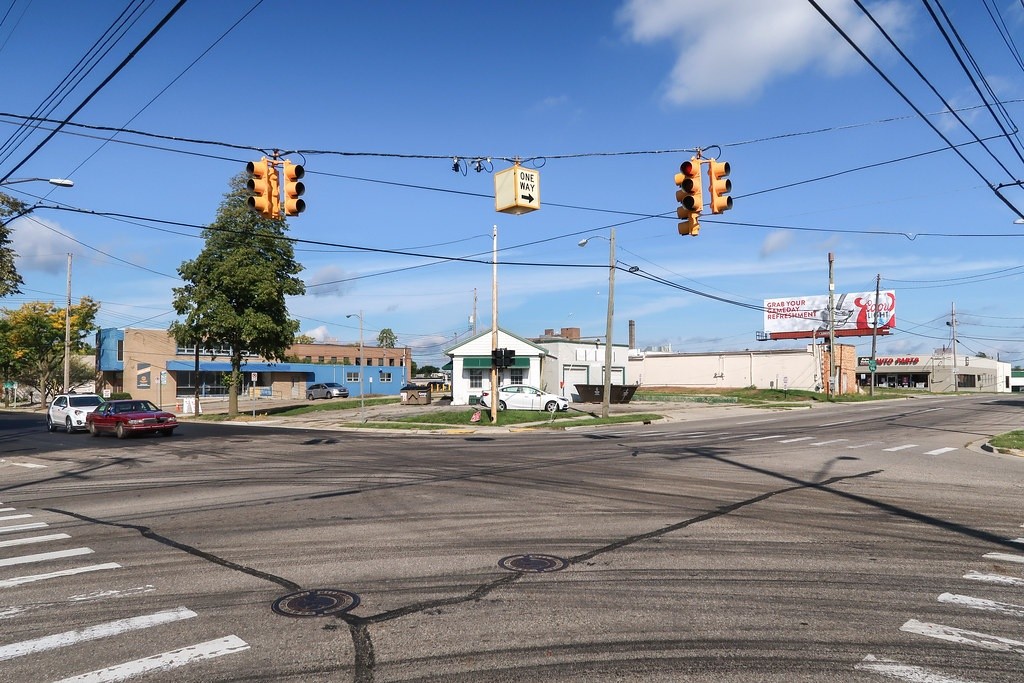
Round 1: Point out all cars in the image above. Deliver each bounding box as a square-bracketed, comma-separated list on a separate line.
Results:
[83, 399, 180, 439]
[46, 394, 112, 434]
[480, 383, 569, 412]
[305, 382, 349, 400]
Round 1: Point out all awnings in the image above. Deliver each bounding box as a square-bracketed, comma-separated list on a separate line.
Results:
[166, 360, 315, 372]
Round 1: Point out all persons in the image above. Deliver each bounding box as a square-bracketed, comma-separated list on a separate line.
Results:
[131, 405, 138, 412]
[107, 404, 112, 414]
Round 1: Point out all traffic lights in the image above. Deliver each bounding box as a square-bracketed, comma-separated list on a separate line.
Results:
[674, 169, 699, 236]
[682, 158, 703, 214]
[248, 155, 270, 215]
[492, 349, 504, 369]
[710, 157, 733, 215]
[282, 160, 306, 217]
[504, 349, 516, 367]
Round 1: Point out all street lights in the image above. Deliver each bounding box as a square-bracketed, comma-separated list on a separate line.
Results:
[345, 309, 366, 423]
[578, 227, 616, 419]
